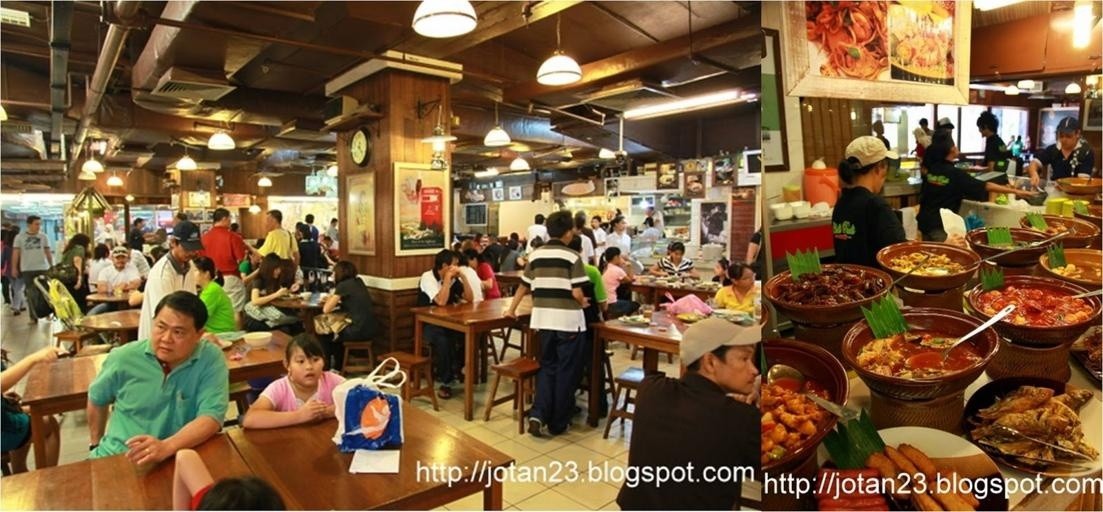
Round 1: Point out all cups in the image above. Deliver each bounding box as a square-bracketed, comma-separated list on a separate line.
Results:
[641, 304, 653, 321]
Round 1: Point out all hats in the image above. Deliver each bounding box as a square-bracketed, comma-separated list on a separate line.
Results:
[1055, 116, 1079, 133]
[844, 136, 900, 169]
[679, 315, 761, 367]
[170, 220, 204, 251]
[110, 246, 129, 257]
[937, 118, 955, 129]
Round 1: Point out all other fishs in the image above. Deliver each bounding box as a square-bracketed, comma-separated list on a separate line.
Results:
[971, 406, 1068, 440]
[1052, 387, 1095, 411]
[977, 384, 1055, 421]
[979, 434, 1061, 471]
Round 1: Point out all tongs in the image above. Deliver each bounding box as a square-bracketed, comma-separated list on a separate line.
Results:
[977, 422, 1095, 470]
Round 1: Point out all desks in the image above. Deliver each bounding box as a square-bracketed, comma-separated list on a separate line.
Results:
[766, 214, 835, 335]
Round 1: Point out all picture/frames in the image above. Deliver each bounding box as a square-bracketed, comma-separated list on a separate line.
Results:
[393, 160, 452, 256]
[779, 2, 971, 108]
[759, 26, 792, 175]
[345, 170, 377, 257]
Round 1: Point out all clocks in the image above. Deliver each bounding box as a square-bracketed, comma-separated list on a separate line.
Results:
[349, 126, 371, 168]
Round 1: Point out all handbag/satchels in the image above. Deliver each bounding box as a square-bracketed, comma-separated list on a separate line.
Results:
[311, 311, 354, 342]
[330, 356, 408, 453]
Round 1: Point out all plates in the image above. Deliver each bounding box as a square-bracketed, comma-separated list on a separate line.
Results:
[1044, 197, 1089, 218]
[887, 4, 954, 79]
[1070, 325, 1103, 381]
[816, 426, 1009, 511]
[617, 316, 650, 326]
[961, 375, 1102, 478]
[637, 272, 719, 290]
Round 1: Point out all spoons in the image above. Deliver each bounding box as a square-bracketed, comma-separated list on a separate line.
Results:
[923, 241, 1041, 276]
[903, 304, 1015, 372]
[766, 364, 853, 420]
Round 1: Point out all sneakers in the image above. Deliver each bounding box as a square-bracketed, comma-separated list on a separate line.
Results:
[527, 416, 545, 437]
[438, 386, 451, 398]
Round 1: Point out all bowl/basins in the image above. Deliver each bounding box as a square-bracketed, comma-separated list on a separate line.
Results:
[243, 331, 272, 350]
[770, 201, 811, 221]
[762, 263, 894, 327]
[759, 337, 849, 472]
[300, 292, 312, 300]
[875, 241, 981, 293]
[967, 275, 1102, 347]
[715, 308, 749, 323]
[1055, 177, 1103, 195]
[1038, 248, 1103, 292]
[964, 227, 1053, 267]
[1071, 205, 1102, 226]
[1019, 213, 1100, 248]
[840, 306, 1000, 400]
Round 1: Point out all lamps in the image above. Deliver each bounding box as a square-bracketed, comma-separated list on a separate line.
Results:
[622, 85, 759, 123]
[1064, 74, 1081, 95]
[415, 93, 458, 153]
[408, 1, 479, 41]
[481, 97, 510, 147]
[72, 128, 271, 216]
[531, 14, 583, 88]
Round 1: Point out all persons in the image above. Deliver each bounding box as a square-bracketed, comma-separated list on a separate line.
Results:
[909, 117, 935, 155]
[873, 119, 890, 152]
[1, 207, 762, 512]
[912, 127, 932, 162]
[1026, 116, 1095, 186]
[1008, 136, 1024, 159]
[914, 138, 1040, 243]
[919, 116, 960, 182]
[975, 110, 1011, 186]
[831, 135, 969, 274]
[1006, 135, 1015, 147]
